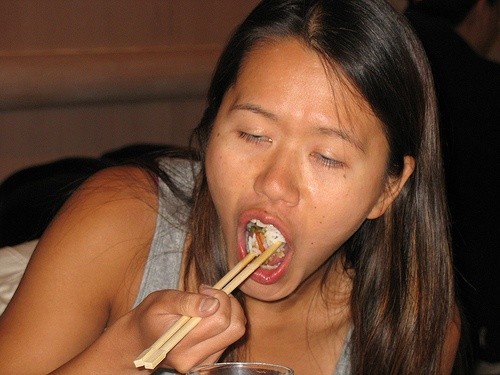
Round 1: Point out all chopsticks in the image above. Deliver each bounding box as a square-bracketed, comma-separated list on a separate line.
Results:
[132, 240, 285, 369]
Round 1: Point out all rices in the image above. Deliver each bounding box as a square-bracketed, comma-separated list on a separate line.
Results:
[246, 223, 286, 257]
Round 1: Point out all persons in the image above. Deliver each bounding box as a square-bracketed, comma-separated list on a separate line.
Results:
[397, 0, 500, 375]
[0, 0, 460, 375]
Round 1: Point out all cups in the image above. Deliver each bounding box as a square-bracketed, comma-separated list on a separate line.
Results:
[186, 363, 294, 375]
